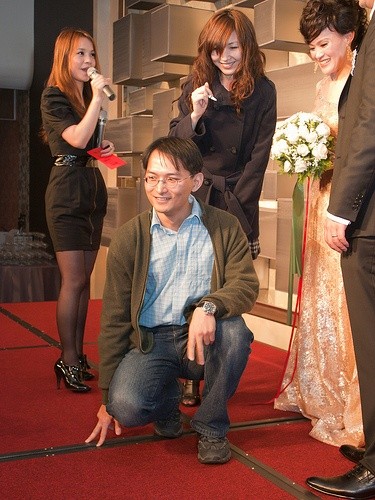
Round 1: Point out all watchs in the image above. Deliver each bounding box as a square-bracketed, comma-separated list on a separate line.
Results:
[196, 301, 216, 315]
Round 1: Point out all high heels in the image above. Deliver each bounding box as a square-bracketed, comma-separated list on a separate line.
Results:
[182, 378, 201, 407]
[78, 355, 94, 381]
[53, 358, 91, 393]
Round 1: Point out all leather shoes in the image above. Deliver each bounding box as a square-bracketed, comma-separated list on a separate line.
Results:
[339, 443, 366, 463]
[304, 462, 375, 500]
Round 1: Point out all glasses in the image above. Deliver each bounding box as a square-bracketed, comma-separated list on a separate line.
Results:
[143, 175, 192, 189]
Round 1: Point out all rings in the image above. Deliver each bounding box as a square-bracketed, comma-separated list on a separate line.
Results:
[110, 146, 112, 148]
[332, 235, 338, 237]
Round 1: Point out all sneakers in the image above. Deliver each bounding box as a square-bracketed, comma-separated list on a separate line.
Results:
[153, 385, 184, 438]
[196, 431, 232, 464]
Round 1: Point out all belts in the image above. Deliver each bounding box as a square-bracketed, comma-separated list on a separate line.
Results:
[51, 154, 99, 168]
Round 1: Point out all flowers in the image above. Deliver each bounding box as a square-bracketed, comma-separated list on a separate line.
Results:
[270, 111, 338, 326]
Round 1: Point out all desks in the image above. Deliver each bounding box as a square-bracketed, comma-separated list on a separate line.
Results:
[0, 265, 61, 303]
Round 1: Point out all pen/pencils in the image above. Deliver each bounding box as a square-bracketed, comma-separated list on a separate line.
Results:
[208, 94, 217, 102]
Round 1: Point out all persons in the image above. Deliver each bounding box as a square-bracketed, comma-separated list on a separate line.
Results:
[305, 0, 375, 500]
[41, 27, 116, 393]
[274, 0, 370, 446]
[85, 136, 260, 465]
[168, 8, 277, 259]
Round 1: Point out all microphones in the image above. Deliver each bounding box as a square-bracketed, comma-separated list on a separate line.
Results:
[87, 67, 116, 101]
